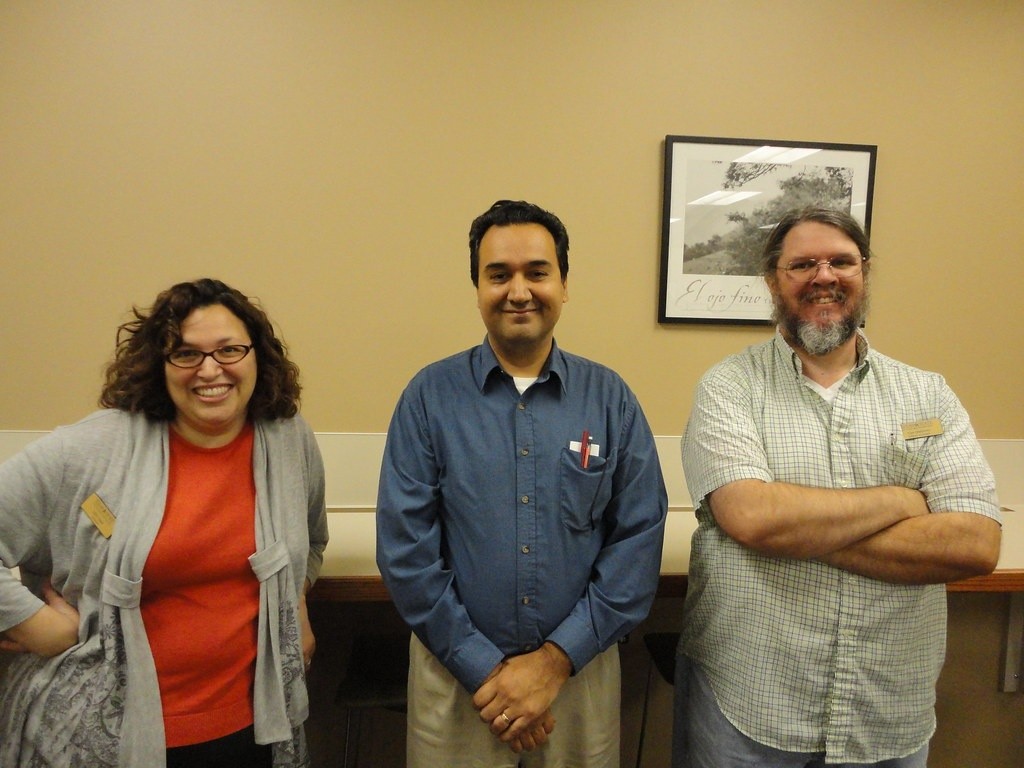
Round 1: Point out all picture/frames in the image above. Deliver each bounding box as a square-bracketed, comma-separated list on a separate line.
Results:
[657, 134, 878, 328]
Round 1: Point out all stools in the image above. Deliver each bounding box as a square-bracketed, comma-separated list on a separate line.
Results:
[341, 634, 409, 768]
[634, 631, 681, 768]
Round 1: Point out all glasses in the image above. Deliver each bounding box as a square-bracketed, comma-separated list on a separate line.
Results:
[163, 341, 256, 369]
[770, 254, 868, 283]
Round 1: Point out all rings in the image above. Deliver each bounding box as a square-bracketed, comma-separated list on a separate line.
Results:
[500, 713, 512, 723]
[307, 659, 311, 666]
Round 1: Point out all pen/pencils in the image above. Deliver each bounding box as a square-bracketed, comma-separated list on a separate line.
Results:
[581, 431, 589, 468]
[890, 433, 895, 450]
[584, 436, 593, 468]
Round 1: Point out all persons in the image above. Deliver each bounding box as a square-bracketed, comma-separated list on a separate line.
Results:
[671, 208, 1003, 768]
[0, 278, 328, 768]
[376, 199, 669, 768]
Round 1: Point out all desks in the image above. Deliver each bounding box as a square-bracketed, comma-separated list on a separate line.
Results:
[0, 430, 1024, 695]
